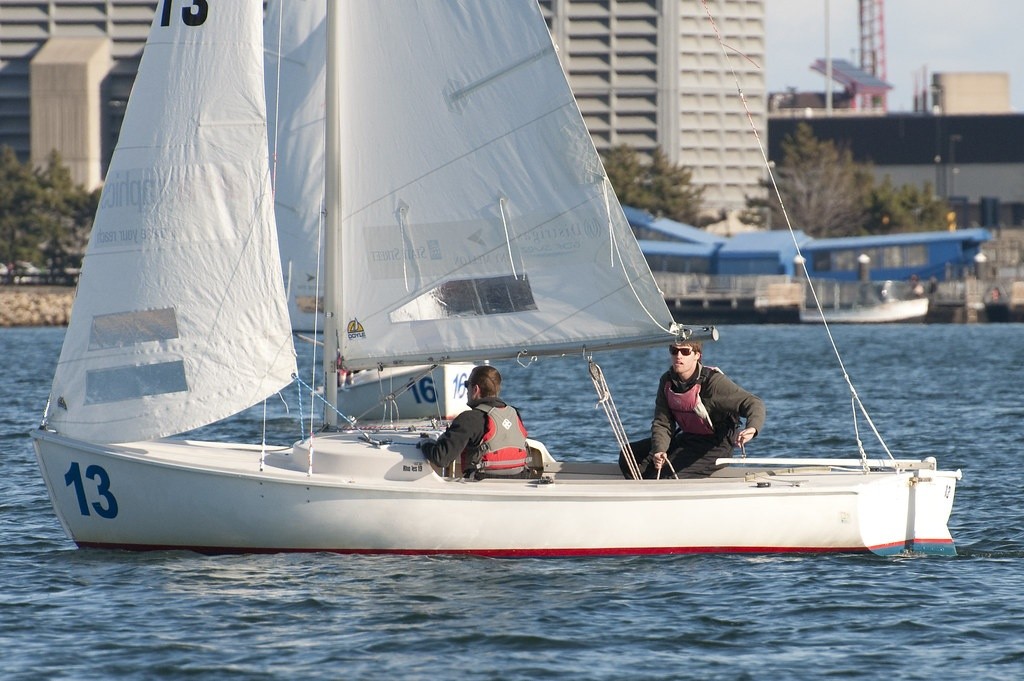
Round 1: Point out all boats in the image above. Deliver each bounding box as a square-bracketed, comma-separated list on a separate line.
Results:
[789, 295, 930, 325]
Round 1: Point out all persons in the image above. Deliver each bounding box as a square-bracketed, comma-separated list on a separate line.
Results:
[617, 338, 765, 481]
[416, 366, 529, 481]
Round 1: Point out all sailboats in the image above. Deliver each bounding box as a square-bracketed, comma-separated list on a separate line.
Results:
[34, 1, 971, 570]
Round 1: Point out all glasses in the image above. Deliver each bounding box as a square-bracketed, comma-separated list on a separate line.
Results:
[464, 380, 474, 388]
[670, 345, 697, 355]
